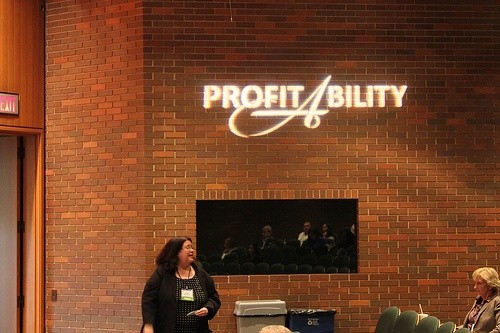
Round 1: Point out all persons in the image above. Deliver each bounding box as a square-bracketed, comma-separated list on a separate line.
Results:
[220, 222, 357, 267]
[140, 236, 221, 333]
[456, 267, 500, 333]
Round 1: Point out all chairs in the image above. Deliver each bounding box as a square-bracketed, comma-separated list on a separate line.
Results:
[373, 307, 470, 333]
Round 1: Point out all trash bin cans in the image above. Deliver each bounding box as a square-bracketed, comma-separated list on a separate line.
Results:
[234, 299, 288, 333]
[286, 307, 336, 333]
